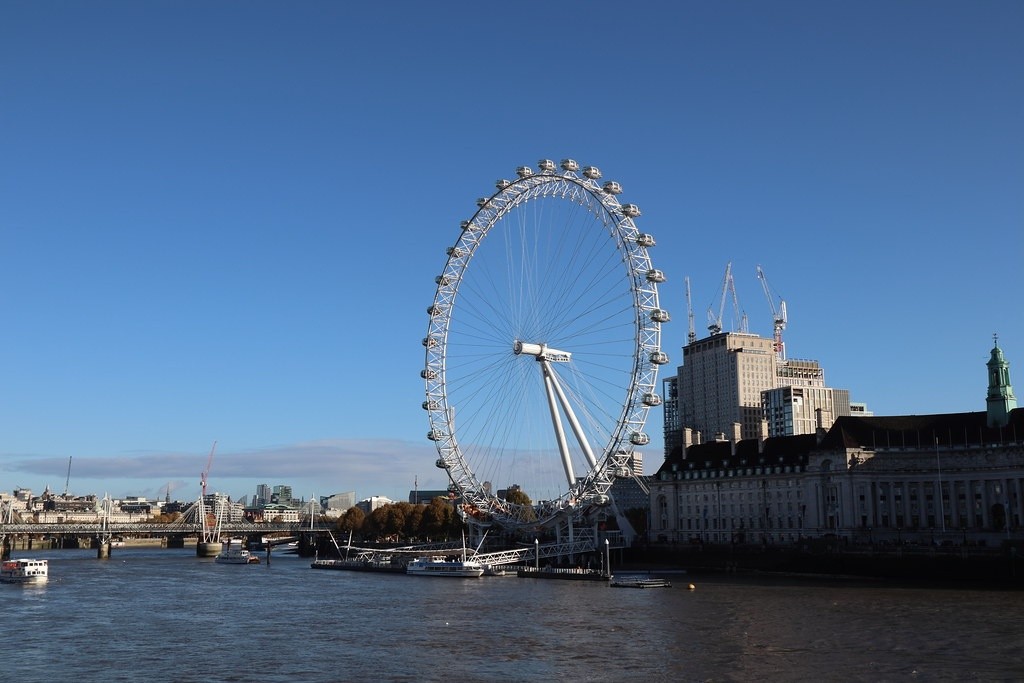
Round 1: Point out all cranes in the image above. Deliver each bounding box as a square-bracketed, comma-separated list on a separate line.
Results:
[200, 440, 216, 496]
[64, 457, 72, 493]
[685, 261, 787, 362]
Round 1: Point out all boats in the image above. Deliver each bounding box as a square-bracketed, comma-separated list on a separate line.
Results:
[214, 550, 261, 564]
[0, 559, 48, 584]
[406, 555, 484, 577]
[311, 551, 391, 570]
